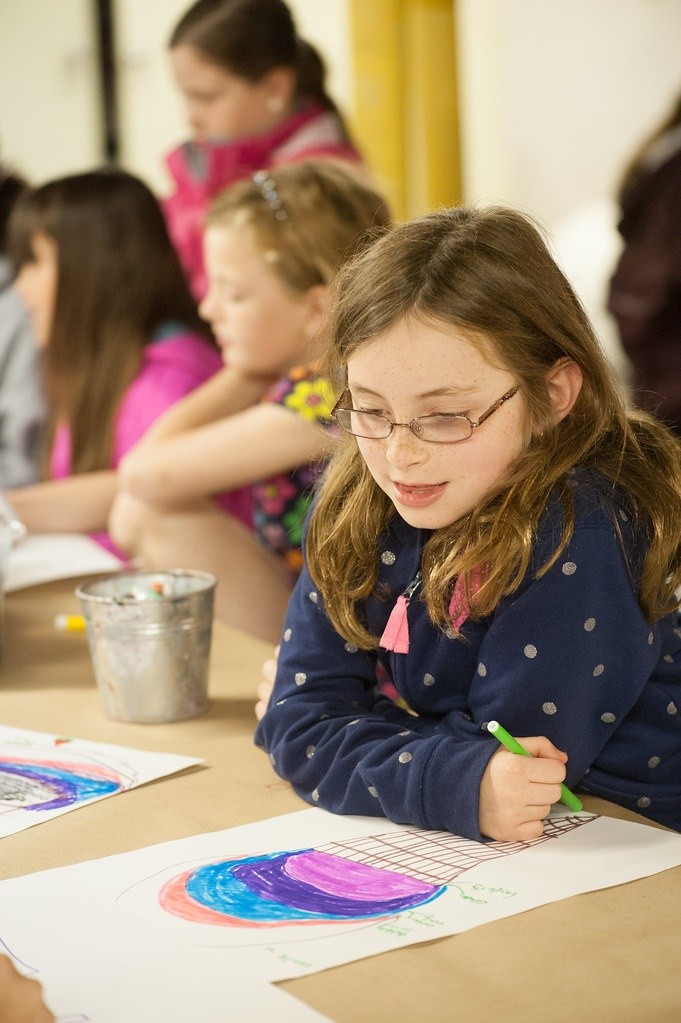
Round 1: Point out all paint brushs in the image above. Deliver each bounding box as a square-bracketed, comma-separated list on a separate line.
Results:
[487, 720, 582, 812]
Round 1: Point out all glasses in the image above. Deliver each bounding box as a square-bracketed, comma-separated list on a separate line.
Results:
[331, 384, 519, 445]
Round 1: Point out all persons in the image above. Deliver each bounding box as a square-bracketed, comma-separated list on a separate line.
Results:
[148, 0, 357, 300]
[246, 205, 681, 842]
[113, 158, 404, 651]
[1, 166, 267, 579]
[1, 176, 63, 478]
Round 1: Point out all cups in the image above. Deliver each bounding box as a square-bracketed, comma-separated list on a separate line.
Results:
[75, 569, 216, 722]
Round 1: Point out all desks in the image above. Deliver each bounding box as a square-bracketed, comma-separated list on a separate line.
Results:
[1, 573, 680, 1023]
